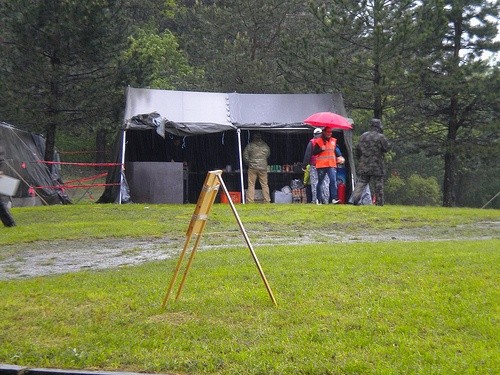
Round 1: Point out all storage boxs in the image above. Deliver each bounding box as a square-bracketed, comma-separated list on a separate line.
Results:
[244, 189, 270, 203]
[272, 191, 292, 203]
[220, 191, 242, 203]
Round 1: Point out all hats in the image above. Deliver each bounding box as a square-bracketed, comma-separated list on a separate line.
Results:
[254, 133, 261, 137]
[313, 128, 322, 133]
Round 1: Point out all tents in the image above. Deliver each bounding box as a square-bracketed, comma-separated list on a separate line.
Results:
[0, 122, 73, 208]
[95, 84, 358, 205]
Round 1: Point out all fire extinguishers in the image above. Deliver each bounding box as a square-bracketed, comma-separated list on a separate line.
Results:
[337, 182, 346, 204]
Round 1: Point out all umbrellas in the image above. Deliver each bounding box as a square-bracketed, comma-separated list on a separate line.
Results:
[304, 110, 352, 147]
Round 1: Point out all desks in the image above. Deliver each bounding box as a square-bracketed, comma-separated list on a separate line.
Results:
[235, 171, 306, 189]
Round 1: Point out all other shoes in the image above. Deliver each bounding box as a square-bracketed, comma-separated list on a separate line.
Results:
[331, 199, 339, 204]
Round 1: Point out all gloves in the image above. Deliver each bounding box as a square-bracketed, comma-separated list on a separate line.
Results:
[302, 168, 305, 172]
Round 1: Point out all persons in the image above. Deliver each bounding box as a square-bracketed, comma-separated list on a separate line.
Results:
[302, 164, 312, 204]
[311, 127, 345, 203]
[302, 128, 331, 206]
[165, 139, 188, 166]
[243, 132, 271, 205]
[348, 119, 391, 206]
